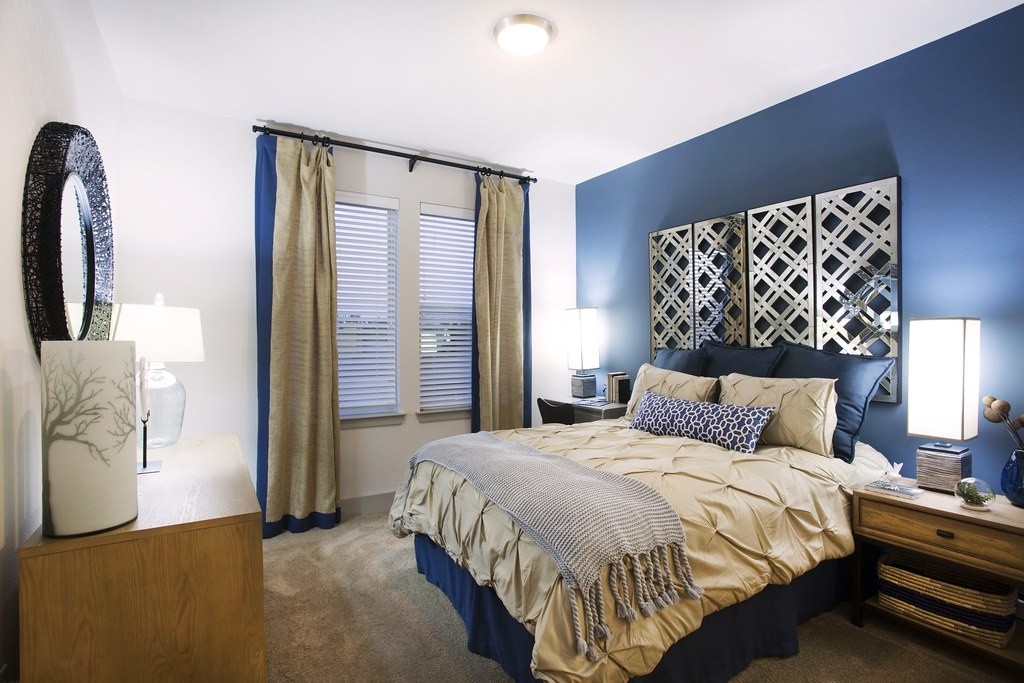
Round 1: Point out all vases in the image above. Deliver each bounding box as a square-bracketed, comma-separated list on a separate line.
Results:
[1000, 450, 1024, 509]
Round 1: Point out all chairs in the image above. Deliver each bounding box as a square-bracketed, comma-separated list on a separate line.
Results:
[537, 397, 574, 424]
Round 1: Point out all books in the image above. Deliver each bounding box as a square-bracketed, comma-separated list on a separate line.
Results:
[864, 479, 925, 499]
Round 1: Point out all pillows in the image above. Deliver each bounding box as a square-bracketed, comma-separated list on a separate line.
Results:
[628, 391, 774, 455]
[769, 341, 896, 464]
[719, 373, 838, 460]
[622, 363, 717, 420]
[653, 346, 707, 377]
[699, 339, 787, 379]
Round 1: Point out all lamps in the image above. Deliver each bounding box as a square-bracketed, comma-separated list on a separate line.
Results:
[562, 307, 602, 398]
[907, 317, 982, 494]
[114, 293, 207, 448]
[492, 15, 554, 58]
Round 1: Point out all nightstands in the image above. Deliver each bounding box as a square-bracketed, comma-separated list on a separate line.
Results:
[851, 478, 1024, 673]
[544, 398, 627, 420]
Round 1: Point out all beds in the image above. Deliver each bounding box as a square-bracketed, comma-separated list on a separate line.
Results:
[387, 417, 904, 682]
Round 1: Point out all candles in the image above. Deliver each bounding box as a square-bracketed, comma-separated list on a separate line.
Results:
[139, 358, 148, 420]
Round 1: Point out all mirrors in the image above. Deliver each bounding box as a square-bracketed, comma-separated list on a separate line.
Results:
[21, 121, 114, 363]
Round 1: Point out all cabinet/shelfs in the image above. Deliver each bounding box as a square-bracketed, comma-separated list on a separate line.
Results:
[20, 434, 266, 683]
